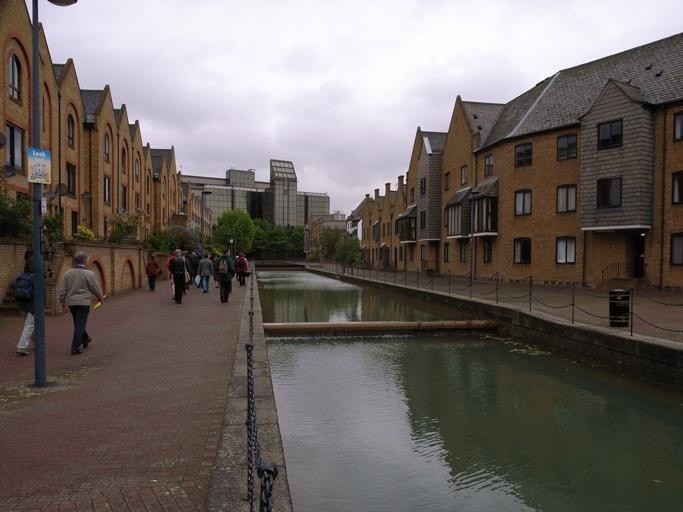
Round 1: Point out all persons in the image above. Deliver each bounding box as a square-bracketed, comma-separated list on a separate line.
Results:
[58, 251, 104, 355]
[146, 254, 159, 291]
[165, 248, 248, 304]
[16, 257, 47, 355]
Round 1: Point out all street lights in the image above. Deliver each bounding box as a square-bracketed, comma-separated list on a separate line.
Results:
[30, 0, 79, 383]
[468, 189, 479, 286]
[200, 189, 211, 252]
[229, 235, 232, 256]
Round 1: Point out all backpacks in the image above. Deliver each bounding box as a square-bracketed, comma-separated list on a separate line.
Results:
[14, 272, 34, 300]
[238, 260, 244, 269]
[217, 258, 228, 274]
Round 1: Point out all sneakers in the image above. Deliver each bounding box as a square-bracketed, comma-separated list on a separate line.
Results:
[83, 336, 92, 348]
[16, 349, 30, 355]
[72, 349, 83, 353]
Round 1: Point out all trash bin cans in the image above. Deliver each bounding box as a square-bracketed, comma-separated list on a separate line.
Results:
[609, 288, 629, 327]
